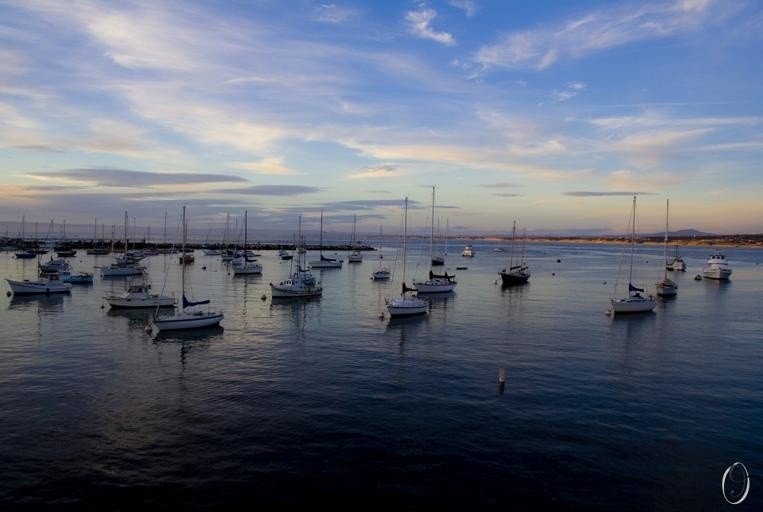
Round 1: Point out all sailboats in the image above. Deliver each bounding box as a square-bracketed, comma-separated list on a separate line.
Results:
[204, 210, 262, 275]
[387, 197, 429, 317]
[4, 216, 109, 297]
[498, 220, 530, 288]
[413, 186, 457, 294]
[657, 199, 677, 298]
[611, 196, 657, 315]
[371, 225, 390, 280]
[100, 206, 224, 338]
[270, 210, 363, 299]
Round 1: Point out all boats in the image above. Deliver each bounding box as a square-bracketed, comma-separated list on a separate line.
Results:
[462, 245, 473, 257]
[703, 251, 732, 280]
[432, 256, 444, 265]
[667, 259, 685, 271]
